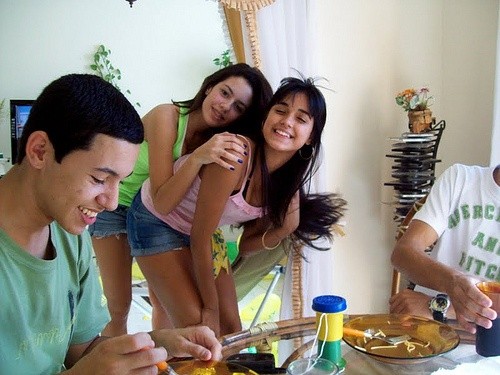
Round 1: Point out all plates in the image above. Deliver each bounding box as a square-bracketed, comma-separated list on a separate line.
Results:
[157, 357, 261, 375]
[343, 313, 460, 364]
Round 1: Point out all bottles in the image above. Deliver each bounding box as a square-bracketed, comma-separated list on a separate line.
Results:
[311, 295, 348, 375]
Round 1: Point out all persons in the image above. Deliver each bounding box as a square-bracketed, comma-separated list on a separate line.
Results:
[388, 158, 500, 335]
[127, 76, 327, 352]
[1, 74, 223, 375]
[87, 63, 275, 336]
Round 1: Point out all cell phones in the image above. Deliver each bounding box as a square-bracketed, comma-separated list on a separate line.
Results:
[225, 353, 287, 375]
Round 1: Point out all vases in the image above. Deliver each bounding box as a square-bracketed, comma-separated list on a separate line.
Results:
[409, 110, 432, 133]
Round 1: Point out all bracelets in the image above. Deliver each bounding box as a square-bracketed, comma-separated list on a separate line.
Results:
[260, 231, 281, 251]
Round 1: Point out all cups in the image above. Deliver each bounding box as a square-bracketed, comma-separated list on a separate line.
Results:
[474, 282, 500, 357]
[286, 358, 339, 375]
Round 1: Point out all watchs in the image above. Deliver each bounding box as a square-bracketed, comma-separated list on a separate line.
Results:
[428, 294, 450, 323]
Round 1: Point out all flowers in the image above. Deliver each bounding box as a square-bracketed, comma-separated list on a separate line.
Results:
[396, 87, 434, 112]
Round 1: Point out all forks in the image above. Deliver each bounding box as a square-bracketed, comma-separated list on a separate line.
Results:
[343, 326, 411, 344]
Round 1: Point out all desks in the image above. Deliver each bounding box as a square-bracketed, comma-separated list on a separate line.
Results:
[157, 315, 500, 375]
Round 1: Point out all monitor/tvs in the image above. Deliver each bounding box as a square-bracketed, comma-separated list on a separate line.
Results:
[10, 100, 39, 163]
